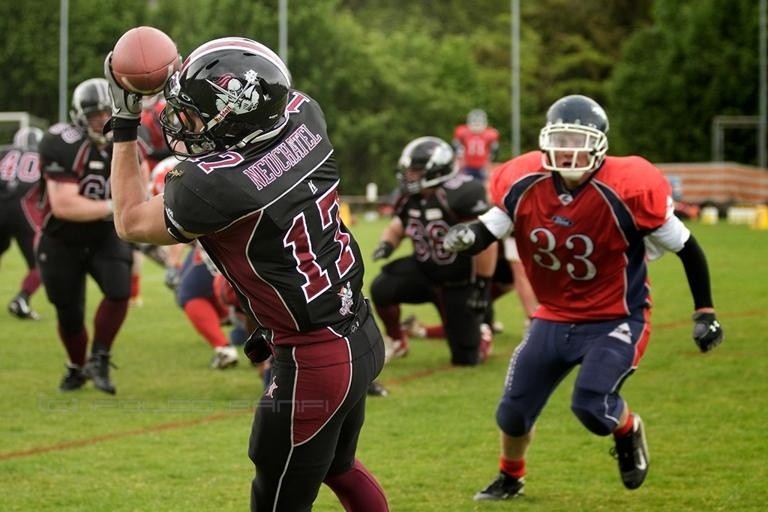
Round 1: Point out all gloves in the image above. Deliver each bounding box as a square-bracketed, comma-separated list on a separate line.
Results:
[466, 276, 492, 313]
[372, 242, 392, 259]
[442, 224, 475, 253]
[691, 311, 722, 353]
[103, 50, 142, 128]
[164, 52, 182, 100]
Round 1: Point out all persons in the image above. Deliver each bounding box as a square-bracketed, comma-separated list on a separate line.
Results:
[367, 135, 502, 369]
[398, 257, 515, 339]
[1, 78, 268, 398]
[451, 109, 500, 183]
[102, 35, 391, 512]
[442, 93, 726, 503]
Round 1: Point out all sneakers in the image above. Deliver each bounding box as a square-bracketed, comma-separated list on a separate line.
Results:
[7, 297, 37, 319]
[472, 469, 524, 501]
[367, 313, 492, 397]
[608, 413, 649, 490]
[60, 363, 86, 390]
[80, 354, 118, 394]
[208, 345, 237, 371]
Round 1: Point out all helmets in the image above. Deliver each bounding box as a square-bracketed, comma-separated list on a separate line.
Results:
[158, 36, 292, 162]
[69, 77, 115, 145]
[13, 126, 44, 152]
[394, 135, 458, 195]
[537, 94, 609, 180]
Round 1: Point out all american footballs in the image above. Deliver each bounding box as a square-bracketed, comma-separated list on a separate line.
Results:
[112, 26, 178, 96]
